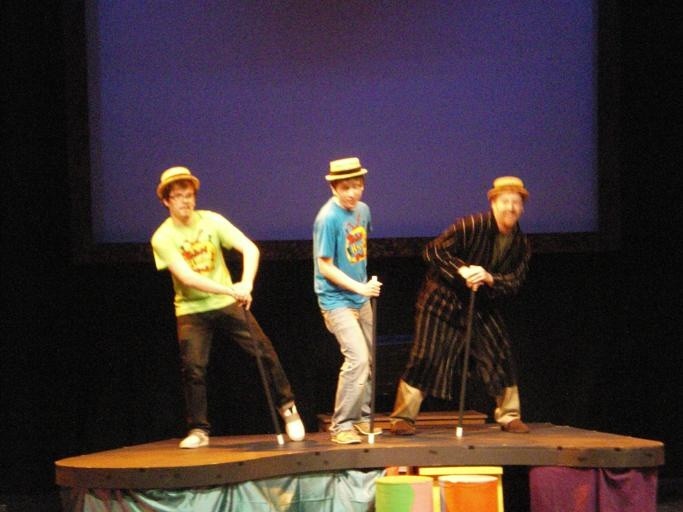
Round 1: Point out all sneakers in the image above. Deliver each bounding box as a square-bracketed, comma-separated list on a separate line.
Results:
[499, 417, 531, 435]
[279, 404, 306, 442]
[177, 428, 210, 450]
[390, 420, 417, 436]
[353, 420, 382, 436]
[330, 430, 362, 444]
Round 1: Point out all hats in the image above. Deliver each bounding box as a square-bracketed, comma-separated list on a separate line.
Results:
[155, 165, 201, 199]
[324, 157, 369, 183]
[486, 175, 529, 201]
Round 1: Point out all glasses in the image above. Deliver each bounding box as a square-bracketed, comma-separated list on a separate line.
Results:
[166, 189, 194, 202]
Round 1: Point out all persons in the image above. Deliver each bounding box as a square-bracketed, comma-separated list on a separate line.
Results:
[309, 156, 383, 446]
[148, 165, 305, 449]
[389, 176, 533, 434]
